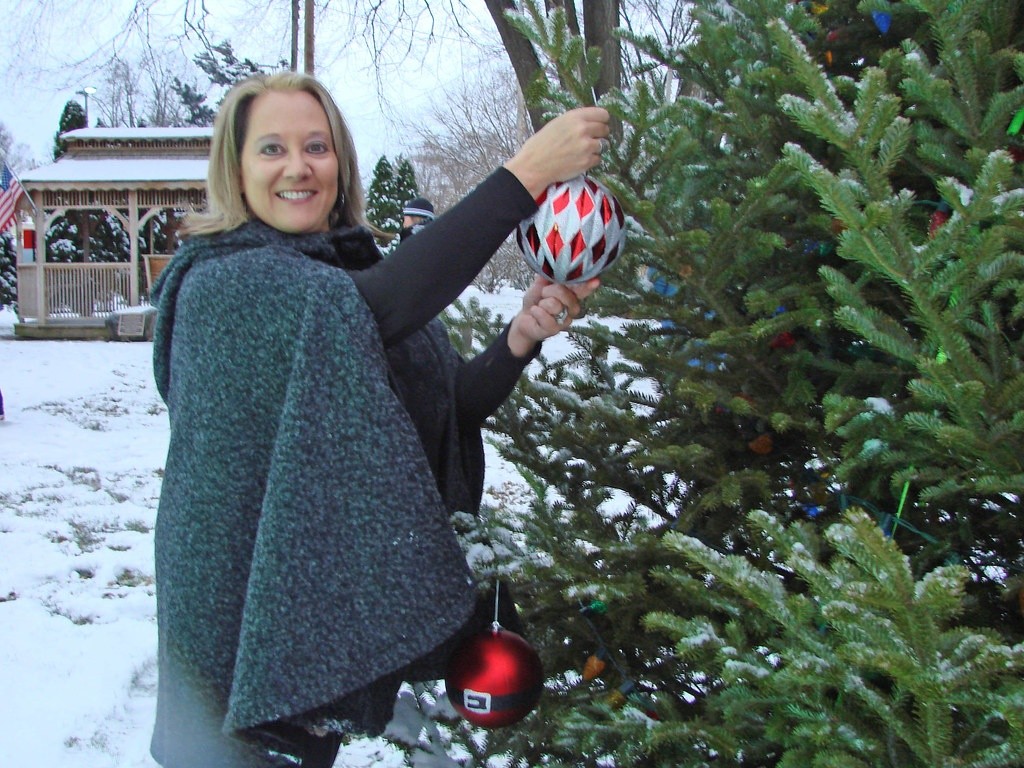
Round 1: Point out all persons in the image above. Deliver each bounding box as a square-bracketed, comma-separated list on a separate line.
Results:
[144, 69, 612, 768]
[398, 197, 436, 245]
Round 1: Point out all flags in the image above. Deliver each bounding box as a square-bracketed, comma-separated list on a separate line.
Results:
[0, 158, 24, 234]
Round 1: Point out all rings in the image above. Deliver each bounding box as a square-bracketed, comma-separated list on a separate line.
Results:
[598, 138, 610, 155]
[554, 305, 569, 326]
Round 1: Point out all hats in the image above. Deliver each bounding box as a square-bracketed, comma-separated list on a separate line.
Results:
[403, 197, 435, 221]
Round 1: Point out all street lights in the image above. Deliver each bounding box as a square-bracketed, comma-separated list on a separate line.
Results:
[76, 87, 96, 129]
